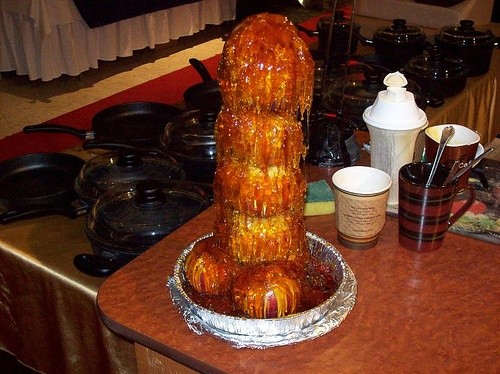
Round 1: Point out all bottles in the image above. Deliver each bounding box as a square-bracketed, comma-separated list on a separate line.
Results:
[362, 71, 427, 207]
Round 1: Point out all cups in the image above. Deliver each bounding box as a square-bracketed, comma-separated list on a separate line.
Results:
[331, 166, 393, 250]
[424, 123, 484, 194]
[398, 161, 477, 255]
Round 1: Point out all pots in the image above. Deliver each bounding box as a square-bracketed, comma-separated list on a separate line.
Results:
[0, 58, 223, 277]
[301, 13, 500, 166]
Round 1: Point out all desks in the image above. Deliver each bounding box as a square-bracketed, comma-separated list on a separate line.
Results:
[0, 0, 500, 374]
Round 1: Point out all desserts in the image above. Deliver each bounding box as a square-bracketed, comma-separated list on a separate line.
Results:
[179, 13, 339, 318]
